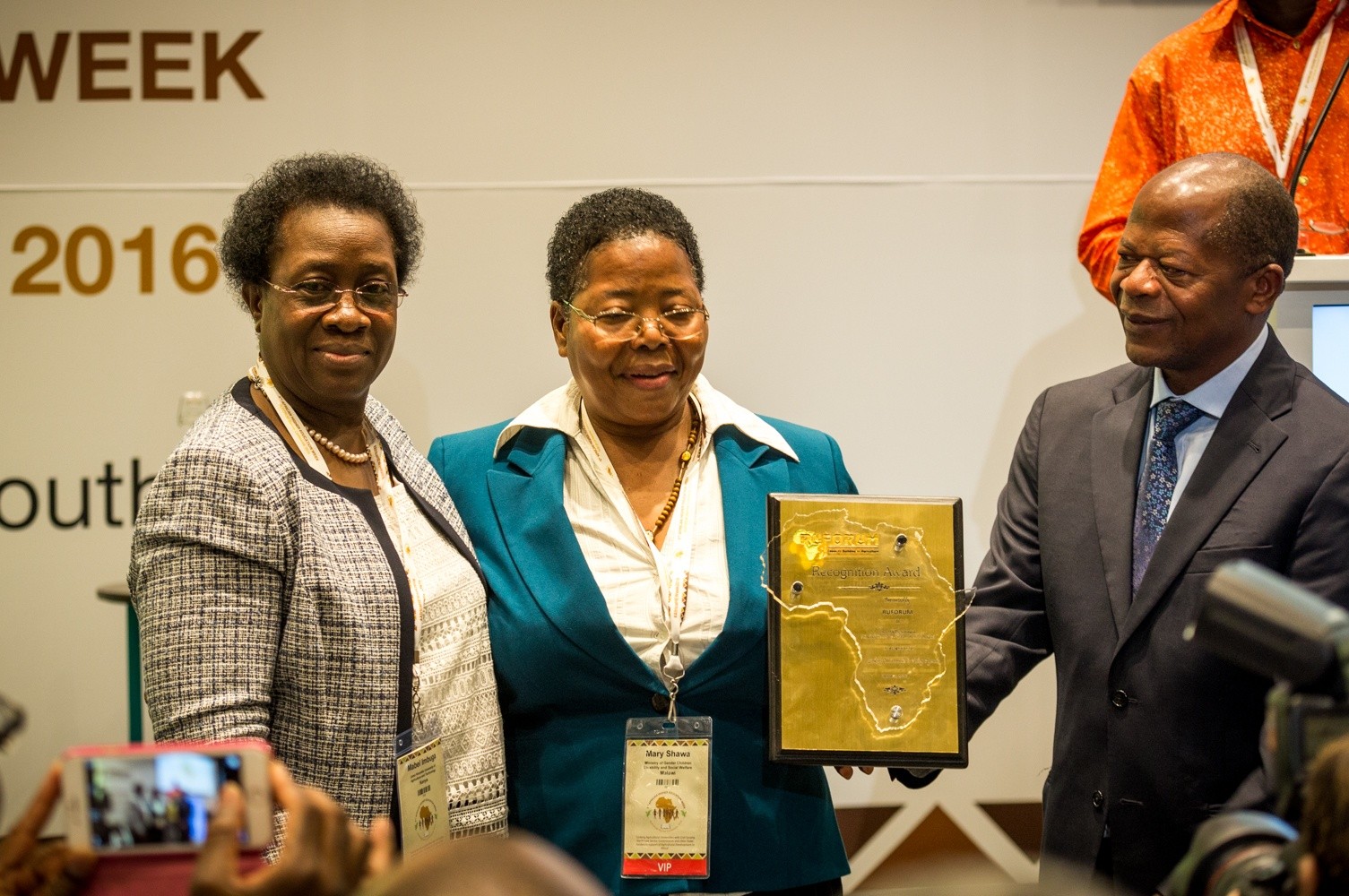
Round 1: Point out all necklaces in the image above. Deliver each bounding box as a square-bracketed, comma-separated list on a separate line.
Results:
[642, 405, 701, 543]
[306, 425, 370, 464]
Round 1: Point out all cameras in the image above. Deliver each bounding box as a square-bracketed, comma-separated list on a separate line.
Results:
[1200, 560, 1349, 789]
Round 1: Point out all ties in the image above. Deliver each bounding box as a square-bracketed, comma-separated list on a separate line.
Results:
[1134, 402, 1204, 598]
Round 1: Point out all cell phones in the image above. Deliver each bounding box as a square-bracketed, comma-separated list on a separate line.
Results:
[63, 736, 274, 861]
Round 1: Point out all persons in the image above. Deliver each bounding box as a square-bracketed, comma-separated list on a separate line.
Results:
[124, 154, 509, 872]
[1079, 0, 1349, 309]
[0, 733, 394, 895]
[89, 765, 194, 840]
[421, 186, 877, 896]
[384, 830, 612, 896]
[877, 154, 1349, 896]
[1279, 734, 1349, 896]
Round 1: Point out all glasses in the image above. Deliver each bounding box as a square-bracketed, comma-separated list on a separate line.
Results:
[560, 297, 710, 341]
[262, 277, 408, 316]
